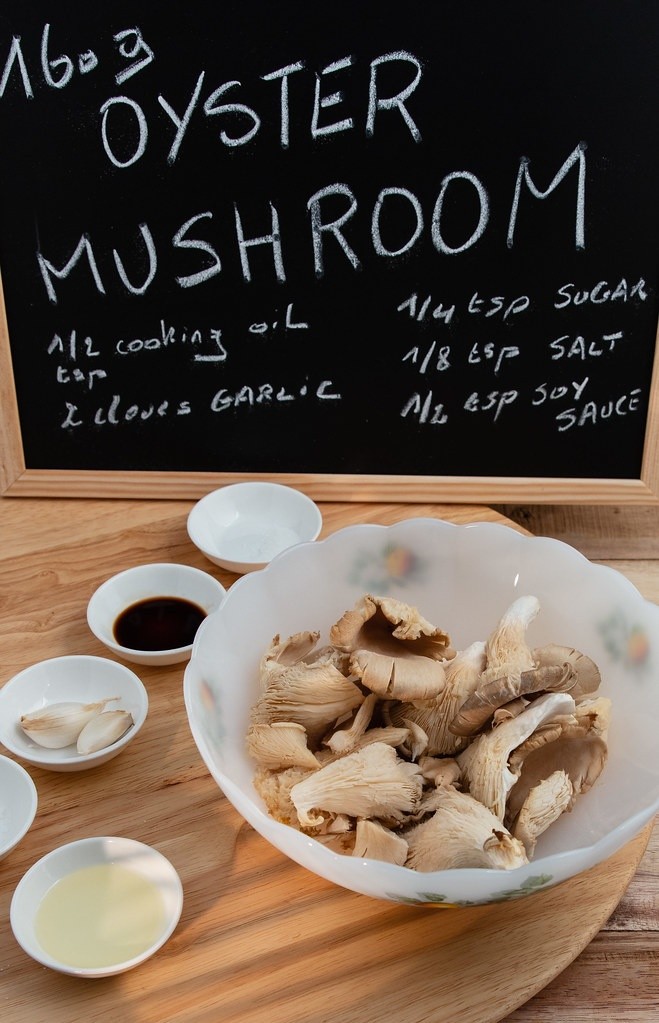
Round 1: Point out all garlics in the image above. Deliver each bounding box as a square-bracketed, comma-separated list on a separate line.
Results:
[18, 701, 133, 757]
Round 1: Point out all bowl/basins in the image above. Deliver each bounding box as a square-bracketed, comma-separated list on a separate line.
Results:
[183, 520, 657, 903]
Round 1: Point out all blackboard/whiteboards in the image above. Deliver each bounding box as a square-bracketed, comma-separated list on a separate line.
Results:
[0, 0, 659, 504]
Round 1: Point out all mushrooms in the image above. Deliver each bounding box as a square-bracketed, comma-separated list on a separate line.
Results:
[246, 594, 610, 873]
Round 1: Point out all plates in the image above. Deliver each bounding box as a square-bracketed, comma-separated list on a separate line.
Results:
[1, 654, 148, 771]
[86, 562, 227, 666]
[0, 755, 38, 858]
[187, 483, 322, 576]
[9, 837, 184, 979]
